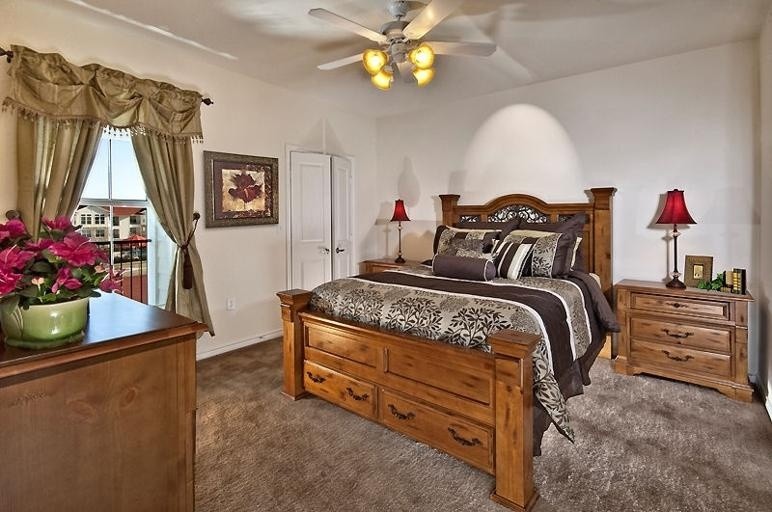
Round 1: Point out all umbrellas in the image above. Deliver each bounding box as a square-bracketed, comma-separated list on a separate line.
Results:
[122, 234, 147, 256]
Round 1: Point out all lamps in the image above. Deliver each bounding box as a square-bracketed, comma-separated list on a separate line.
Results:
[389, 198, 411, 264]
[362, 43, 437, 90]
[654, 189, 697, 289]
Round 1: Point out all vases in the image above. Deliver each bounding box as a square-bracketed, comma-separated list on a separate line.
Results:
[3, 296, 90, 349]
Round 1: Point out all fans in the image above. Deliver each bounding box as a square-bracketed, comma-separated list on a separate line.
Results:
[308, 0, 496, 90]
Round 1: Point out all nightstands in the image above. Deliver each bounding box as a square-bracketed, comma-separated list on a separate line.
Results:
[614, 277, 754, 404]
[363, 257, 414, 272]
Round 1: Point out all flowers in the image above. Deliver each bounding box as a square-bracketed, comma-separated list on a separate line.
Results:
[0, 209, 128, 315]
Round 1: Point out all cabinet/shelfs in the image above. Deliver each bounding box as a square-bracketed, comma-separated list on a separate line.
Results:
[1, 288, 209, 510]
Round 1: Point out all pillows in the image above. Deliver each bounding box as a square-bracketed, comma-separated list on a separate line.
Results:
[491, 239, 536, 280]
[518, 212, 587, 279]
[502, 229, 563, 279]
[436, 229, 500, 256]
[453, 218, 519, 241]
[437, 237, 488, 259]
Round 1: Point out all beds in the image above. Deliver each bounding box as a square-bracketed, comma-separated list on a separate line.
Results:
[275, 186, 618, 512]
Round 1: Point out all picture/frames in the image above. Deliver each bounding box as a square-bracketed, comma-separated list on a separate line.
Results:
[685, 255, 713, 289]
[202, 150, 279, 228]
